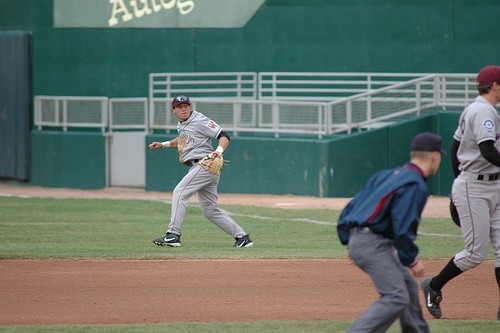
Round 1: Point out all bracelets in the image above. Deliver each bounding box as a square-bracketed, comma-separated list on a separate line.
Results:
[216, 146, 224, 153]
[161, 141, 171, 148]
[410, 261, 418, 268]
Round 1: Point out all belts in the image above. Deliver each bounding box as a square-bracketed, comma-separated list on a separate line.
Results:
[477, 172, 500, 180]
[348, 228, 375, 235]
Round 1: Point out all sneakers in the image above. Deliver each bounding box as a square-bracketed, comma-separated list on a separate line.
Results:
[234, 232, 254, 249]
[153, 233, 181, 247]
[421, 277, 442, 319]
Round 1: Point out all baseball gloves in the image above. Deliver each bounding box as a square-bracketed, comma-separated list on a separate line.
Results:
[195, 152, 224, 177]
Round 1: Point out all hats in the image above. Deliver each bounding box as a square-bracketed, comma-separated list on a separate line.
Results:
[408, 131, 448, 156]
[172, 95, 191, 109]
[476, 65, 500, 89]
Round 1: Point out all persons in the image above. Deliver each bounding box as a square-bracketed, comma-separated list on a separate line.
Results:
[420, 65, 500, 321]
[149, 95, 254, 248]
[336, 132, 449, 333]
